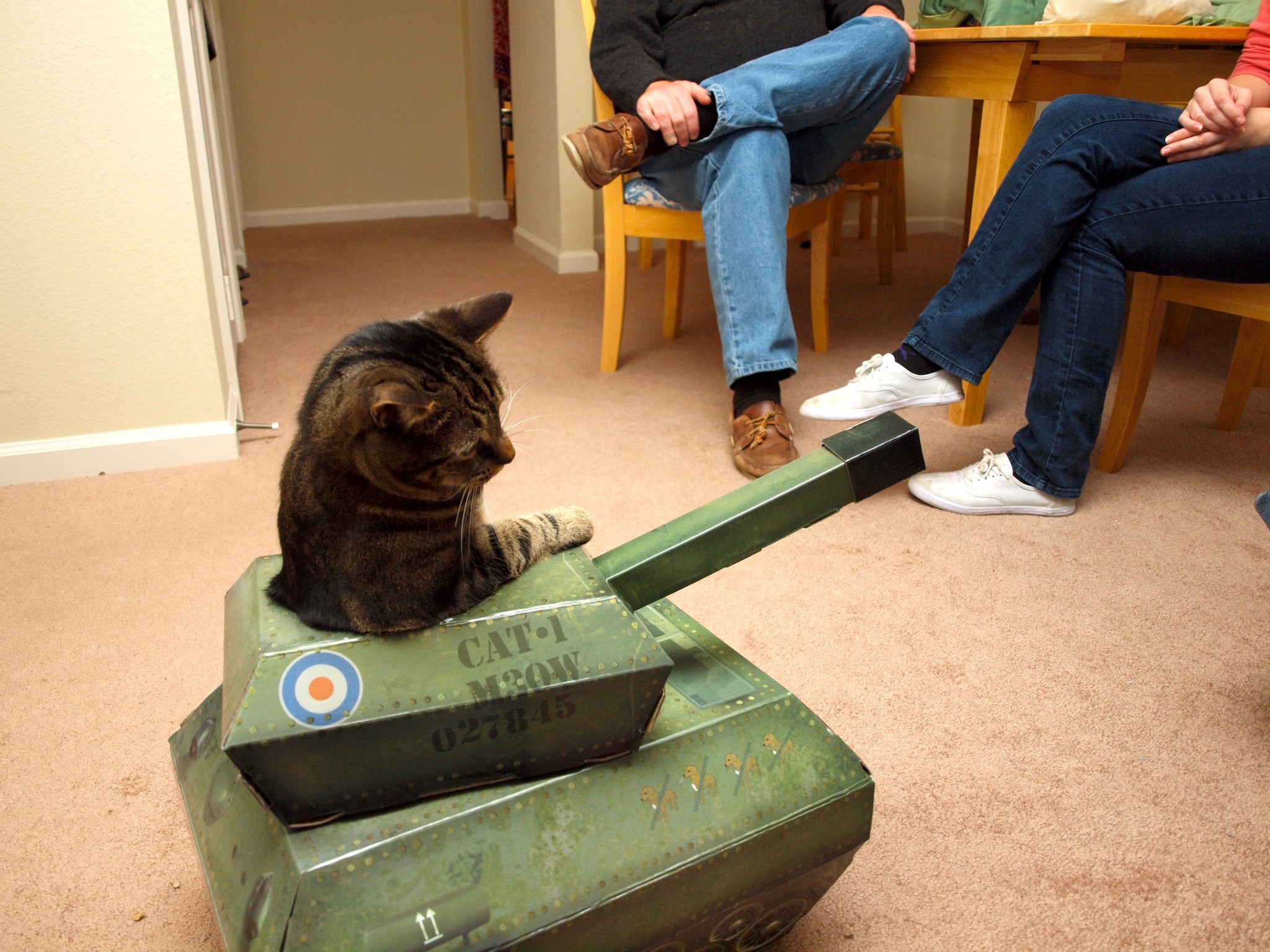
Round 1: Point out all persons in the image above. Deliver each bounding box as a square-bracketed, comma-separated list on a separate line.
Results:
[799, 1, 1270, 522]
[558, 1, 921, 485]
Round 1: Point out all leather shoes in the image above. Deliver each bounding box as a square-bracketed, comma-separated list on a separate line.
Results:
[729, 392, 799, 480]
[562, 111, 647, 191]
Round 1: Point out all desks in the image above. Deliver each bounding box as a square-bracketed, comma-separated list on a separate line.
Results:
[906, 21, 1256, 428]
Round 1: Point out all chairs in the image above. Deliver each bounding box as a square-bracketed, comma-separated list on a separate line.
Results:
[585, 1, 849, 374]
[1094, 265, 1270, 475]
[832, 86, 912, 291]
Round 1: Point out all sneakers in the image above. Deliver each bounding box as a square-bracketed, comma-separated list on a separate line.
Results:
[800, 353, 967, 422]
[906, 451, 1076, 516]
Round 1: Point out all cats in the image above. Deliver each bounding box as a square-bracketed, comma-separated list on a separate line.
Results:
[260, 292, 592, 637]
